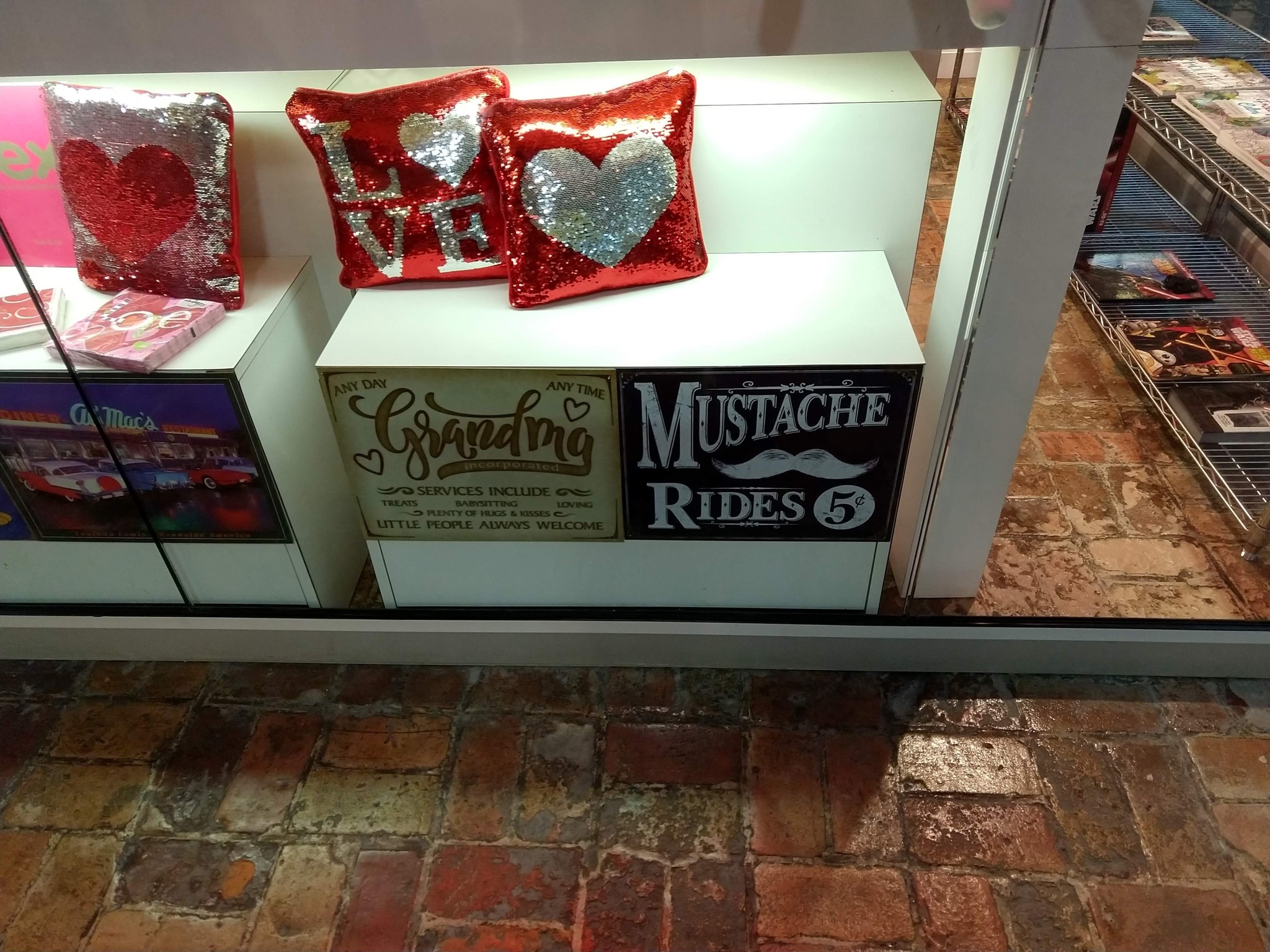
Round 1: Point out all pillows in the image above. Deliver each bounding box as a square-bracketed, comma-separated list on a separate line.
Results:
[284, 68, 508, 289]
[482, 67, 709, 307]
[39, 81, 245, 310]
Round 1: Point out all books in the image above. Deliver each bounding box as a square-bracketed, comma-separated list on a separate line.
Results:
[1141, 15, 1201, 46]
[1114, 315, 1270, 381]
[1073, 249, 1217, 302]
[1132, 55, 1270, 183]
[1166, 382, 1270, 444]
